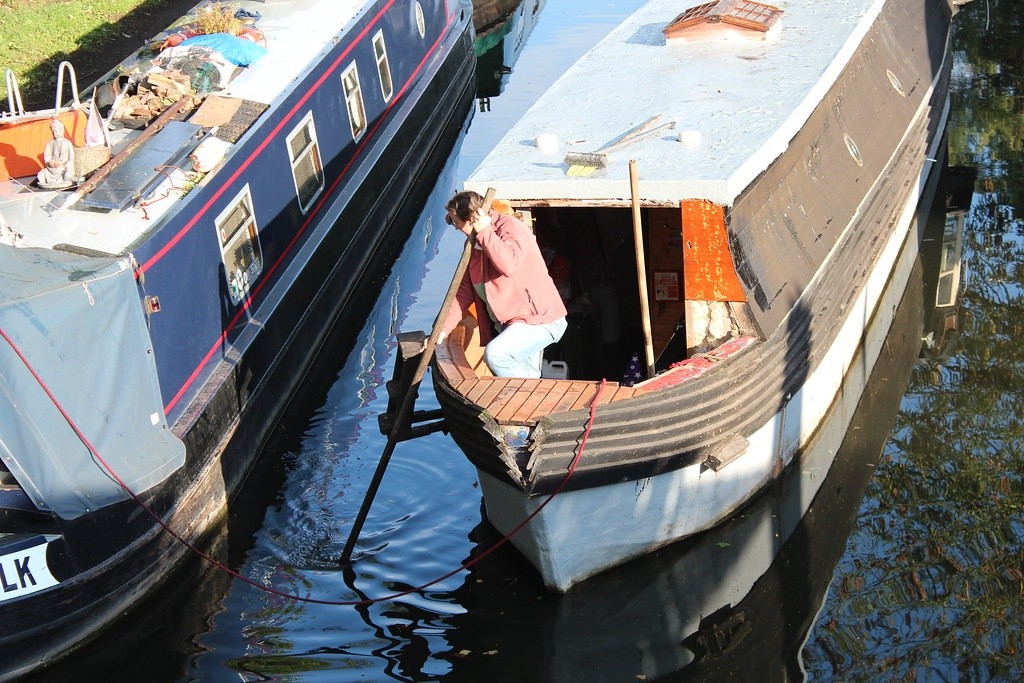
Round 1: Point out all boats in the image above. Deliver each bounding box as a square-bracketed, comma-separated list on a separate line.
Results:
[0, 0, 477, 683]
[427, 0, 954, 596]
[458, 127, 978, 683]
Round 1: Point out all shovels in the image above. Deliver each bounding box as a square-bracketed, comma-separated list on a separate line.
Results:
[566, 112, 662, 176]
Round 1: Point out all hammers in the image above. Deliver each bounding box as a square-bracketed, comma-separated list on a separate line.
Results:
[564, 121, 677, 165]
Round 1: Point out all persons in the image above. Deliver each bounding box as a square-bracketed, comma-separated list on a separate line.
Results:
[420, 191, 568, 378]
[38, 120, 74, 183]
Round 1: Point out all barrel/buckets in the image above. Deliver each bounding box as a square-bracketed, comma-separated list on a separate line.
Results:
[541, 358, 569, 380]
